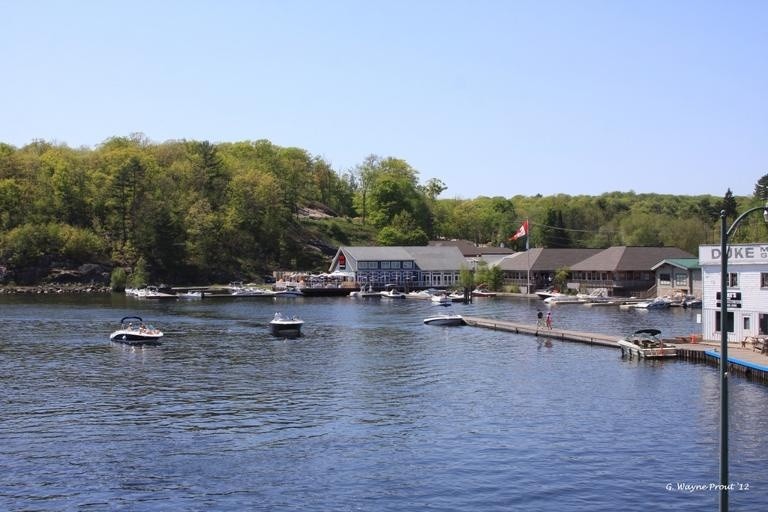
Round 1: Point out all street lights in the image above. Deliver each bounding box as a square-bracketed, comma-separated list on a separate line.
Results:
[718, 206, 768, 511]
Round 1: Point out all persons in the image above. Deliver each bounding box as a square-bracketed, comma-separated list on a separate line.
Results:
[273, 312, 282, 321]
[536, 308, 547, 330]
[545, 311, 552, 330]
[125, 322, 160, 335]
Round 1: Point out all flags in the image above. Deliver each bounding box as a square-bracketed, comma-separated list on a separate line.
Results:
[508, 219, 529, 242]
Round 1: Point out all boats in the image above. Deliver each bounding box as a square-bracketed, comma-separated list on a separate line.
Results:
[125, 288, 213, 301]
[424, 315, 463, 326]
[109, 327, 164, 345]
[616, 329, 679, 358]
[269, 317, 305, 337]
[231, 272, 496, 306]
[536, 288, 701, 310]
